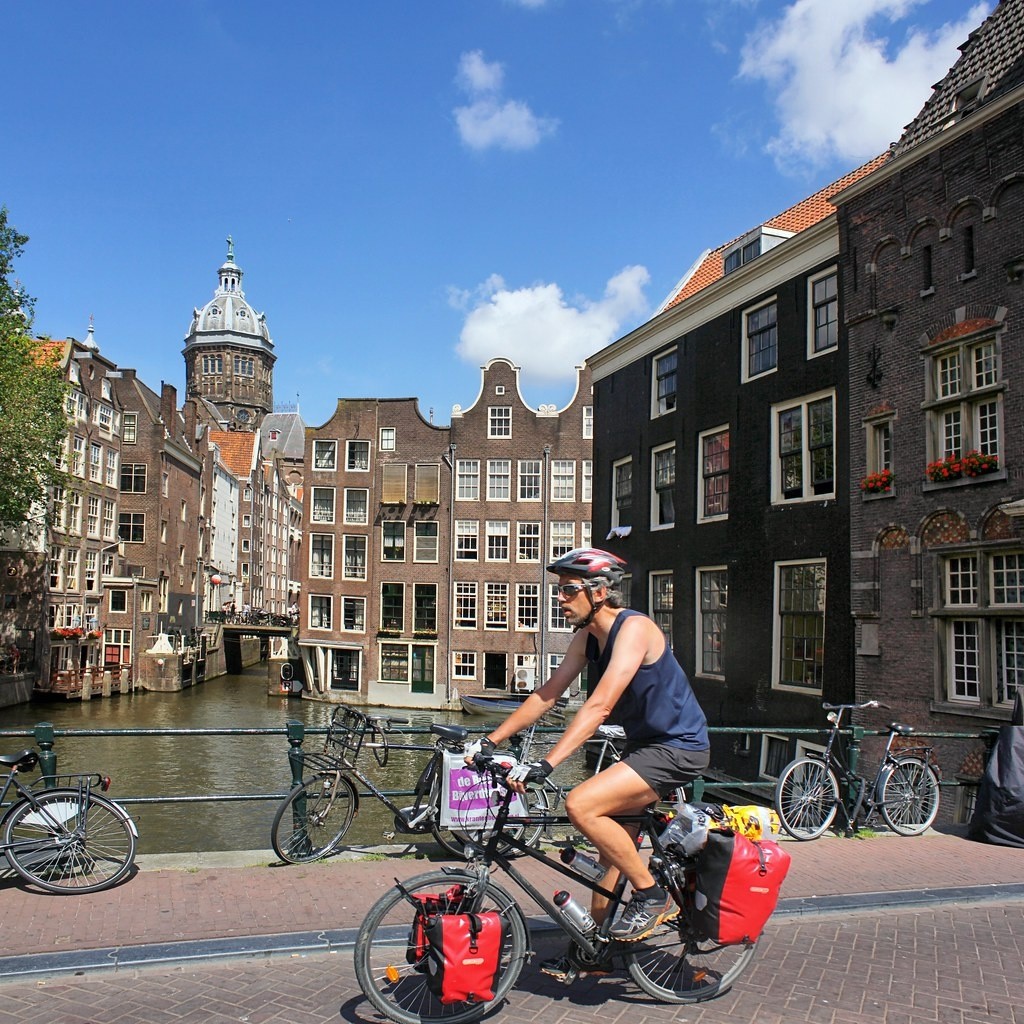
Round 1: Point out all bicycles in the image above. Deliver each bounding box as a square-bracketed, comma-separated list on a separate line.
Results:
[351, 755, 765, 1024]
[776, 699, 942, 842]
[452, 721, 688, 857]
[269, 704, 531, 864]
[0, 748, 142, 896]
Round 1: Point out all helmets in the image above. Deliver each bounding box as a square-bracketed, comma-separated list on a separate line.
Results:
[546, 548, 628, 586]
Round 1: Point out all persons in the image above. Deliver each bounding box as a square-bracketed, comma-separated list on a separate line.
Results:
[291, 602, 299, 627]
[460, 545, 714, 979]
[242, 601, 252, 625]
[221, 598, 237, 625]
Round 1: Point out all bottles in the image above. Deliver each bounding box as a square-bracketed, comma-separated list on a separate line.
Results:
[558, 847, 603, 879]
[553, 891, 593, 931]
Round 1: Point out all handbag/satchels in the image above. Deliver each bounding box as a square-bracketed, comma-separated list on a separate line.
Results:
[439, 749, 528, 831]
[406, 884, 511, 1006]
[692, 825, 791, 945]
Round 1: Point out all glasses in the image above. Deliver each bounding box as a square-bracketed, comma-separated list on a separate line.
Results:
[558, 582, 598, 596]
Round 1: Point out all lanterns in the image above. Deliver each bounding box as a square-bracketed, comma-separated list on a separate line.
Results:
[211, 574, 222, 585]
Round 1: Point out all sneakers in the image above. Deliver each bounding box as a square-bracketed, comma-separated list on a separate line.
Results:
[540, 935, 614, 977]
[609, 888, 681, 942]
